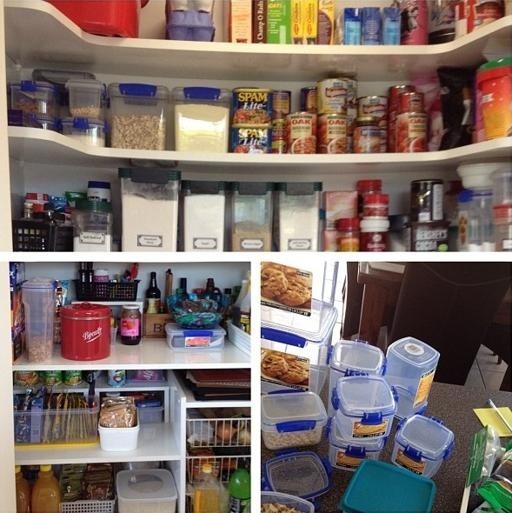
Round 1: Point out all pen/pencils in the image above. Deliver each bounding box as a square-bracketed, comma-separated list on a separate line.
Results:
[487, 398, 512, 435]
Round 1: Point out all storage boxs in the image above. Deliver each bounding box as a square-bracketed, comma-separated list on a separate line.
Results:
[275, 181, 323, 252]
[136, 399, 165, 423]
[8, 80, 60, 115]
[43, 0, 151, 38]
[164, 322, 226, 353]
[15, 396, 101, 444]
[171, 86, 233, 153]
[225, 318, 252, 358]
[12, 219, 74, 253]
[70, 199, 114, 253]
[326, 339, 387, 418]
[118, 167, 180, 252]
[97, 407, 139, 451]
[454, 156, 511, 192]
[457, 189, 493, 252]
[57, 301, 112, 361]
[260, 299, 340, 397]
[324, 414, 388, 474]
[71, 279, 141, 301]
[59, 498, 115, 513]
[383, 336, 441, 421]
[389, 413, 455, 481]
[64, 78, 106, 117]
[107, 82, 170, 151]
[331, 376, 398, 442]
[230, 181, 274, 251]
[261, 391, 329, 451]
[260, 490, 316, 513]
[181, 180, 229, 252]
[21, 279, 58, 364]
[489, 166, 512, 206]
[123, 370, 167, 387]
[60, 117, 110, 149]
[116, 469, 178, 513]
[7, 109, 62, 137]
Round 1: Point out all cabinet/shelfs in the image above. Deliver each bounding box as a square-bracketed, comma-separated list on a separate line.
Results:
[11, 322, 256, 513]
[1, 0, 512, 254]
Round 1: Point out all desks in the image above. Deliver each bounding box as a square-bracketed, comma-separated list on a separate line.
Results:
[356, 261, 408, 347]
[353, 262, 357, 268]
[256, 363, 512, 512]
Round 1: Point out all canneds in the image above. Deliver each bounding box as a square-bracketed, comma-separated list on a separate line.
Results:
[400, 92, 423, 112]
[231, 129, 272, 154]
[285, 111, 317, 154]
[357, 96, 388, 152]
[318, 114, 352, 154]
[331, 76, 359, 120]
[353, 117, 382, 154]
[299, 86, 318, 113]
[396, 113, 428, 151]
[230, 87, 273, 128]
[271, 91, 291, 120]
[388, 85, 415, 153]
[316, 77, 347, 115]
[273, 119, 287, 153]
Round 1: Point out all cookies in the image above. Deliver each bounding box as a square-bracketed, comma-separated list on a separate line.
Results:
[267, 350, 297, 365]
[281, 361, 308, 383]
[262, 355, 288, 377]
[275, 278, 310, 306]
[261, 269, 288, 298]
[263, 262, 298, 283]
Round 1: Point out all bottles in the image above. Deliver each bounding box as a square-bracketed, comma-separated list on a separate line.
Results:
[16, 203, 40, 249]
[229, 460, 250, 513]
[337, 219, 360, 252]
[180, 277, 188, 300]
[232, 285, 241, 304]
[164, 268, 173, 313]
[219, 288, 233, 340]
[411, 179, 444, 222]
[120, 305, 142, 345]
[32, 465, 60, 513]
[15, 465, 30, 513]
[206, 278, 214, 300]
[176, 288, 184, 301]
[356, 179, 382, 219]
[145, 272, 161, 313]
[363, 195, 389, 219]
[490, 167, 512, 206]
[454, 190, 483, 251]
[387, 214, 411, 251]
[193, 464, 222, 512]
[238, 310, 249, 335]
[7, 64, 22, 126]
[360, 220, 390, 252]
[231, 280, 249, 326]
